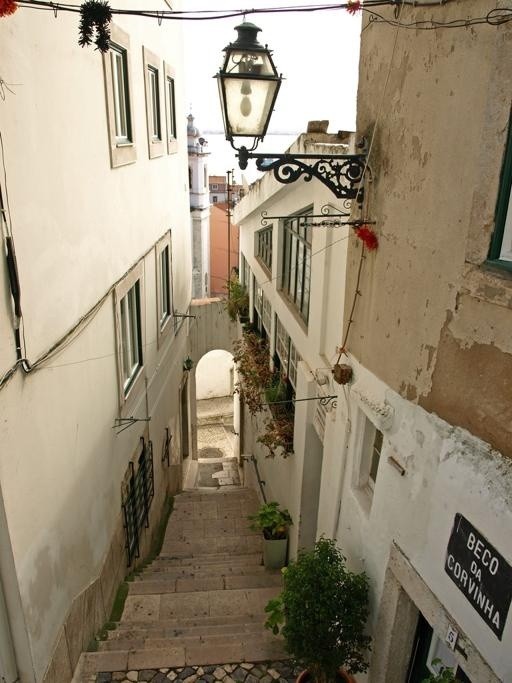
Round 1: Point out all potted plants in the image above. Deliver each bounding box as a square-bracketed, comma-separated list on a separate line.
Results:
[264, 531, 374, 683]
[245, 501, 293, 568]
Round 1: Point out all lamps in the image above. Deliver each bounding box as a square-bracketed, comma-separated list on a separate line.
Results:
[212, 22, 371, 226]
